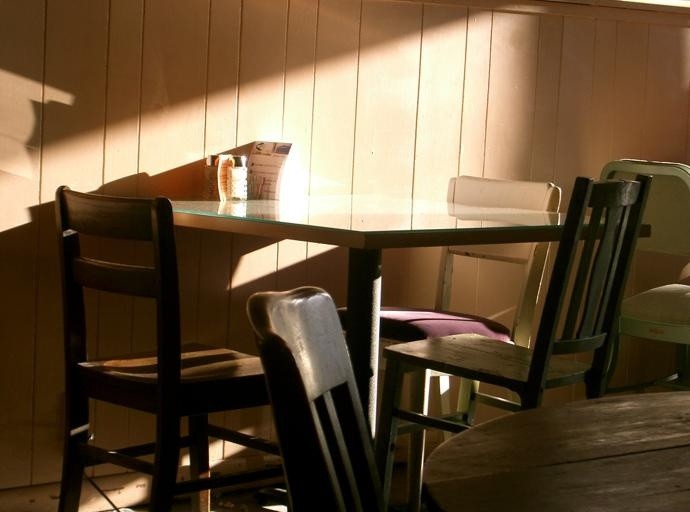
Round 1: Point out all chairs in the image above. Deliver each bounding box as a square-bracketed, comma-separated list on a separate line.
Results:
[246, 286, 394, 510]
[598, 160, 689, 284]
[618, 283, 689, 346]
[378, 175, 651, 498]
[54, 186, 290, 511]
[341, 175, 561, 439]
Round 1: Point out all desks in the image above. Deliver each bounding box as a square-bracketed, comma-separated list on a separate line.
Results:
[423, 391, 688, 510]
[168, 200, 651, 440]
